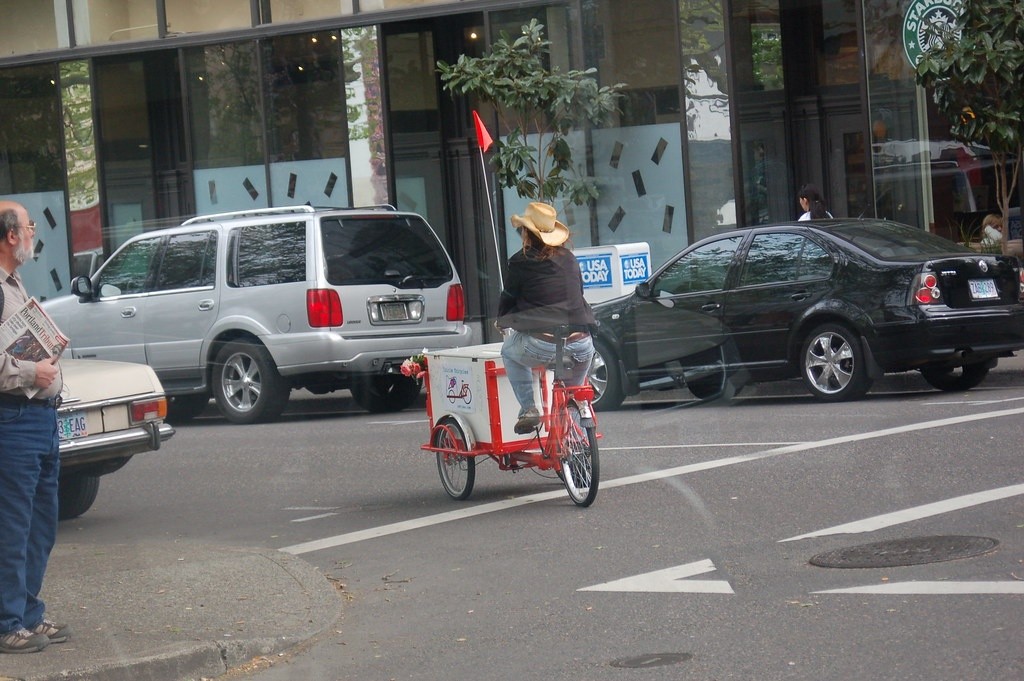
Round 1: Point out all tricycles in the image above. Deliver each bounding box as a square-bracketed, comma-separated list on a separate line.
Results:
[420, 325, 603, 509]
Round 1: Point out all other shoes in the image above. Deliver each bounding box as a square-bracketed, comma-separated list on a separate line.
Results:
[513, 408, 540, 435]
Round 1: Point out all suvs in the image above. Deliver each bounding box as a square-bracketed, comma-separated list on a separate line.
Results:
[37, 202, 474, 427]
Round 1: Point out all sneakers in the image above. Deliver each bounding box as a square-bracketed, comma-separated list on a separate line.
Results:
[30, 619, 74, 644]
[0, 627, 51, 654]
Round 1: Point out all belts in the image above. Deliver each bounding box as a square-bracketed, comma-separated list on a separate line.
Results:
[530, 331, 590, 347]
[0, 394, 63, 410]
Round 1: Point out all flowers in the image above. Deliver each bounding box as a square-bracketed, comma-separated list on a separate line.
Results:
[401, 347, 429, 389]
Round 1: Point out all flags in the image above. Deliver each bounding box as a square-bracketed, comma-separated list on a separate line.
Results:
[472, 110, 493, 152]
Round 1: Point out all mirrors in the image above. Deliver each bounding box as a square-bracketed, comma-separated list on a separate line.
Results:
[57, 59, 107, 280]
[674, 0, 745, 247]
[338, 25, 398, 212]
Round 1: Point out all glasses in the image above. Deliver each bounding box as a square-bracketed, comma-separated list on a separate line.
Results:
[516, 226, 524, 235]
[14, 219, 36, 231]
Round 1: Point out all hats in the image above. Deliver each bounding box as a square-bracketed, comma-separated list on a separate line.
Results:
[510, 202, 569, 247]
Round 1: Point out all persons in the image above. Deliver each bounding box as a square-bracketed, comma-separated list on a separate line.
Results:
[982, 215, 1003, 243]
[798, 183, 834, 222]
[0, 200, 68, 654]
[495, 201, 598, 466]
[10, 334, 45, 363]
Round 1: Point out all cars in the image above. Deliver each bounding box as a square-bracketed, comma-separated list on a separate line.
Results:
[586, 218, 1023, 413]
[48, 360, 174, 523]
